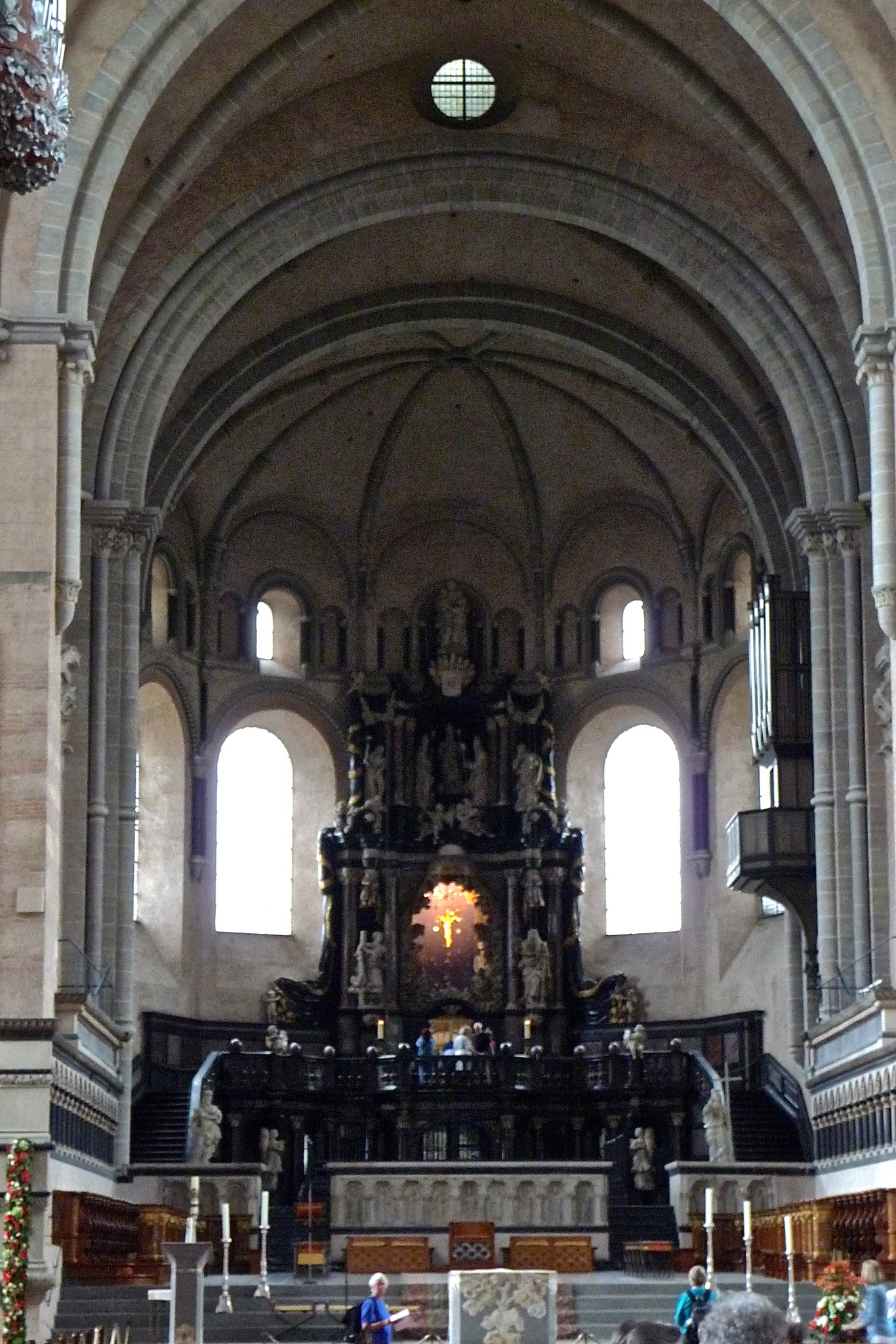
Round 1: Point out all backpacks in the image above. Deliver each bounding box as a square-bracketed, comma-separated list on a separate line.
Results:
[686, 1288, 711, 1344]
[422, 1041, 432, 1057]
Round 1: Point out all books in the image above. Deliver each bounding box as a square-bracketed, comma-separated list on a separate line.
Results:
[390, 1309, 409, 1322]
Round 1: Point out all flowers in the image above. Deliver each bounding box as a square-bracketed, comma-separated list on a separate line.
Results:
[0, 1139, 35, 1344]
[805, 1259, 865, 1335]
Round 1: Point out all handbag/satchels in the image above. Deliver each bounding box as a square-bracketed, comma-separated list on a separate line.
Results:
[339, 1297, 378, 1342]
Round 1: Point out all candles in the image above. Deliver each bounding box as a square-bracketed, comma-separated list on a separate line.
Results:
[742, 1200, 753, 1237]
[524, 1019, 532, 1039]
[377, 1019, 384, 1039]
[784, 1212, 794, 1252]
[260, 1190, 269, 1227]
[705, 1187, 714, 1224]
[190, 1174, 200, 1188]
[220, 1200, 230, 1240]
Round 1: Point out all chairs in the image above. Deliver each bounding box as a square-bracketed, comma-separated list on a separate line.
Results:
[447, 1221, 494, 1271]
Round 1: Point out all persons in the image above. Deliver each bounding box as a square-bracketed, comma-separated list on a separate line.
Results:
[440, 1031, 454, 1070]
[264, 1025, 280, 1050]
[189, 1088, 222, 1164]
[363, 744, 386, 801]
[673, 1265, 716, 1344]
[703, 1087, 730, 1164]
[471, 1022, 495, 1086]
[414, 800, 496, 842]
[261, 1129, 285, 1192]
[513, 744, 543, 812]
[629, 1024, 645, 1060]
[417, 1028, 435, 1083]
[463, 738, 495, 807]
[886, 1288, 896, 1330]
[517, 930, 552, 1009]
[631, 1127, 656, 1191]
[264, 989, 281, 1024]
[841, 1259, 893, 1344]
[435, 581, 469, 663]
[361, 1273, 393, 1344]
[447, 1027, 472, 1072]
[362, 931, 387, 990]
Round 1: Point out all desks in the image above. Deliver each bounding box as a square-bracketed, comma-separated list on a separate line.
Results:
[270, 1304, 420, 1340]
[509, 1236, 593, 1274]
[623, 1240, 673, 1280]
[346, 1236, 431, 1274]
